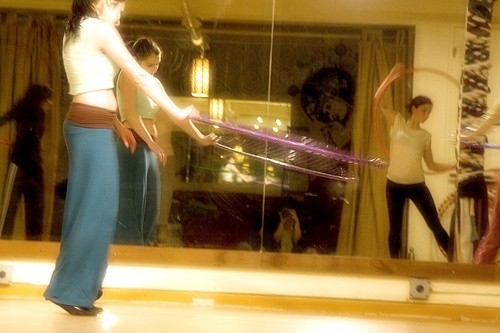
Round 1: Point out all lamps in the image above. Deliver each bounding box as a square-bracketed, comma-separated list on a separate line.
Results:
[191, 44, 209, 97]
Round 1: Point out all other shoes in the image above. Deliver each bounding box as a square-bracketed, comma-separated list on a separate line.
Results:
[87, 304, 103, 314]
[45, 298, 97, 317]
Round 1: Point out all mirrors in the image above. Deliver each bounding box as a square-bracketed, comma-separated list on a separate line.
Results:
[0, 0, 500, 282]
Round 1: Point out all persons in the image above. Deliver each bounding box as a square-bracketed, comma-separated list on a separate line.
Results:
[233, 206, 323, 253]
[115, 36, 222, 246]
[372, 60, 460, 262]
[457, 88, 498, 264]
[43, 0, 197, 319]
[0, 82, 56, 241]
